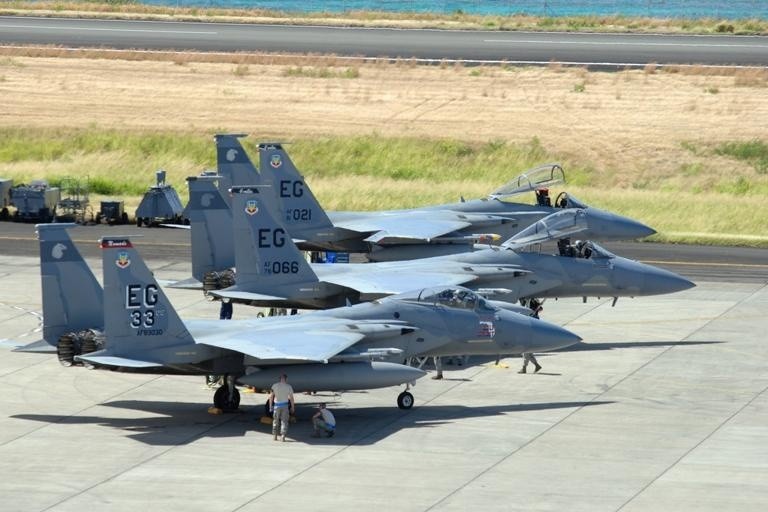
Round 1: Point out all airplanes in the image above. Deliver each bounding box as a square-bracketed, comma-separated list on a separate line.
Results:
[164, 176, 697, 317]
[183, 132, 660, 262]
[9, 221, 584, 422]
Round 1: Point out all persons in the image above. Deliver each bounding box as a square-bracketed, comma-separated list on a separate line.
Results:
[574, 240, 589, 258]
[311, 402, 336, 438]
[517, 352, 541, 374]
[519, 298, 541, 319]
[268, 374, 295, 441]
